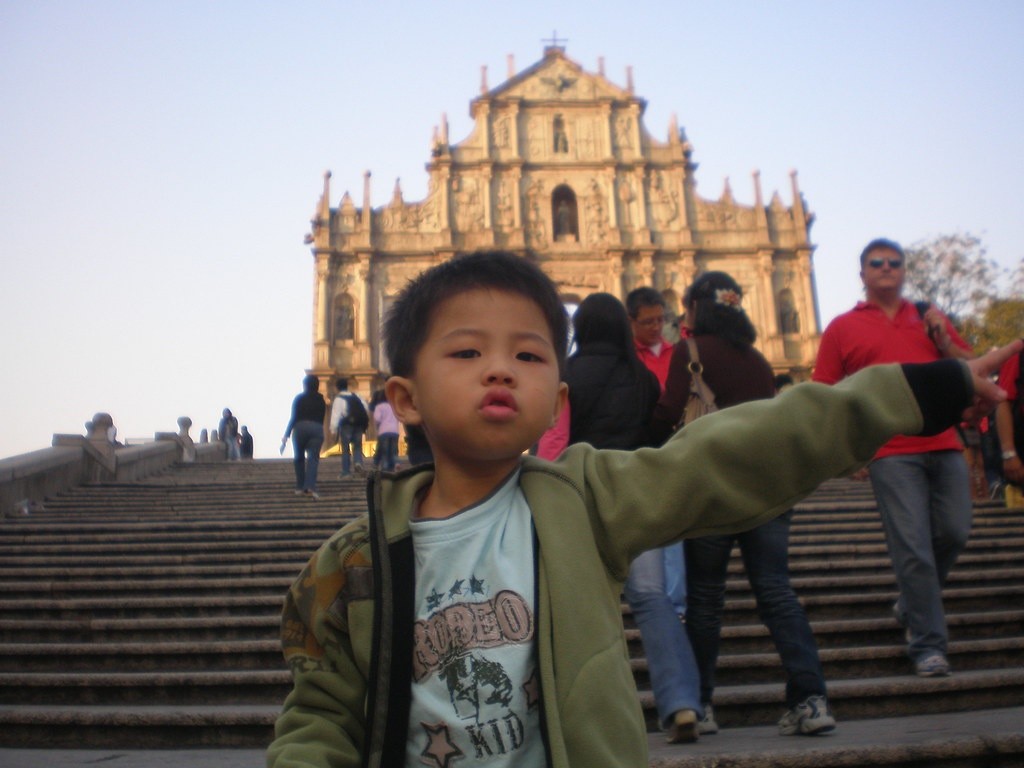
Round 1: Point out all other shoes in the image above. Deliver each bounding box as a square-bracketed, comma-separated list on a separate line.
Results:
[294, 489, 319, 498]
[988, 480, 1004, 500]
[667, 709, 699, 742]
[337, 474, 353, 480]
[355, 463, 367, 476]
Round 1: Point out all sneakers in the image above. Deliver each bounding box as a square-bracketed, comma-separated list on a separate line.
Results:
[917, 652, 952, 676]
[699, 704, 718, 733]
[893, 602, 912, 643]
[777, 696, 837, 736]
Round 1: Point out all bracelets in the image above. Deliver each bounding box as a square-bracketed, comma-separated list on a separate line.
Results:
[1001, 449, 1015, 460]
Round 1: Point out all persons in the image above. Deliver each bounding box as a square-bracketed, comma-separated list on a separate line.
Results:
[266, 248, 1023, 768]
[370, 376, 400, 470]
[281, 375, 326, 502]
[367, 393, 404, 473]
[963, 340, 1024, 507]
[329, 378, 369, 482]
[811, 237, 972, 677]
[218, 408, 254, 463]
[540, 272, 838, 746]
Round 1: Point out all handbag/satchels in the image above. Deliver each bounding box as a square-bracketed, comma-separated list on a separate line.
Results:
[675, 336, 720, 429]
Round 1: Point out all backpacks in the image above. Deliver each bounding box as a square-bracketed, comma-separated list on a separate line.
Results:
[336, 390, 370, 430]
[913, 301, 995, 450]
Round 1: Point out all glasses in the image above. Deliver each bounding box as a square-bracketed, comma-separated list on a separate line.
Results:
[632, 316, 668, 328]
[865, 257, 905, 268]
